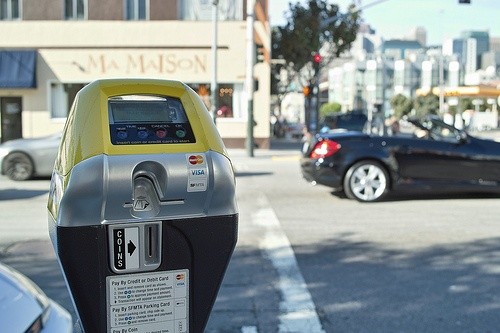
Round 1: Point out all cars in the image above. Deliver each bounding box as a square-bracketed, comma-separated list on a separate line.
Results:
[290, 123, 306, 138]
[321, 113, 367, 133]
[0, 128, 64, 182]
[299, 115, 500, 202]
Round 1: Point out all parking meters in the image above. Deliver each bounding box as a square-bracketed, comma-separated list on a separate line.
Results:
[47, 79, 238, 333]
